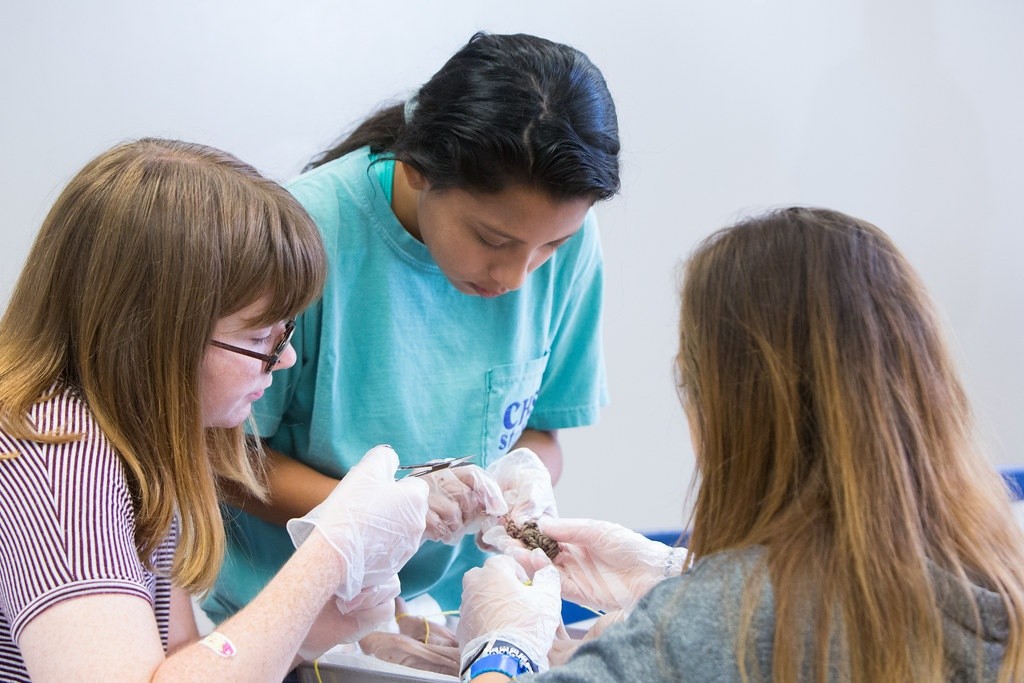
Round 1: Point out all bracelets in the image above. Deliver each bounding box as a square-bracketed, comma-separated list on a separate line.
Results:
[663, 547, 675, 581]
[458, 640, 539, 682]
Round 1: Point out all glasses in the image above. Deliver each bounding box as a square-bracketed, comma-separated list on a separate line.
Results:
[204, 319, 297, 374]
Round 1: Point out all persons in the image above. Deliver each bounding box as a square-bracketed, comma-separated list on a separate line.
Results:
[0, 138, 431, 682]
[188, 31, 620, 640]
[453, 204, 1023, 682]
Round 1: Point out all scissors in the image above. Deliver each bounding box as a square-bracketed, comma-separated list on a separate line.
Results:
[396, 453, 478, 477]
